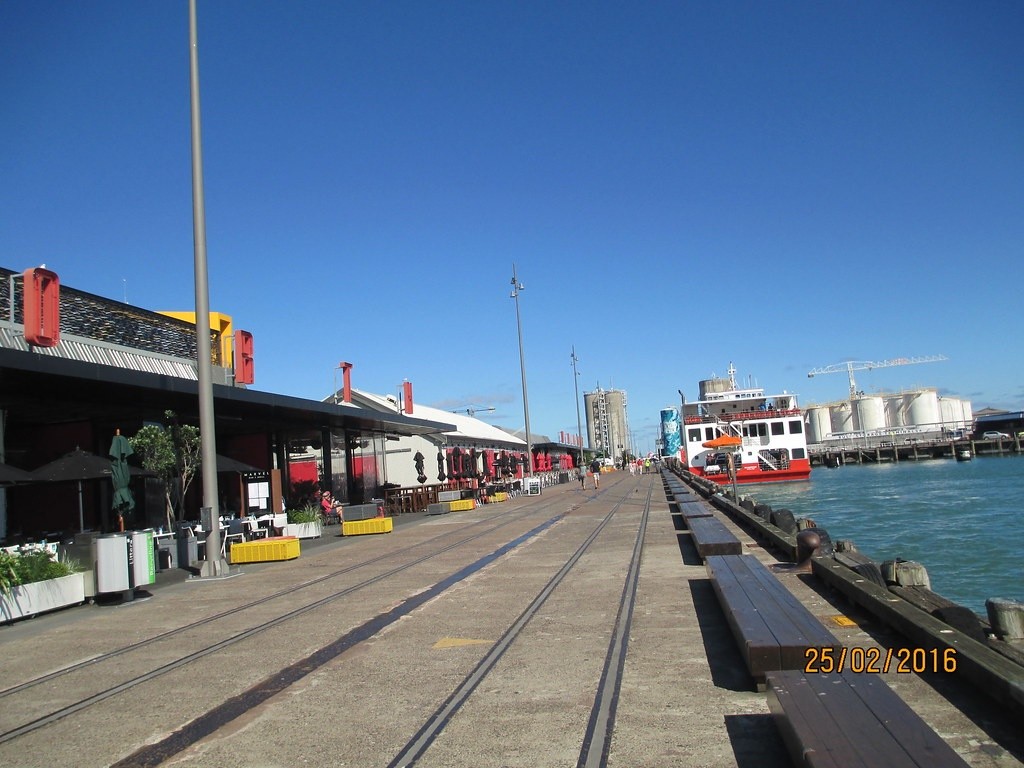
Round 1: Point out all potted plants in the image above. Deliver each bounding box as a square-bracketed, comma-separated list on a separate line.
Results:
[0, 547, 89, 622]
[286, 499, 327, 538]
[127, 409, 202, 569]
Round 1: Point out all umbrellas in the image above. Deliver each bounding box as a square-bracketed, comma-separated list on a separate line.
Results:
[0, 446, 268, 535]
[109, 430, 135, 534]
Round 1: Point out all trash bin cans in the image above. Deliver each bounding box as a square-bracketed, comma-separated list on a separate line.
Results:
[91, 528, 156, 593]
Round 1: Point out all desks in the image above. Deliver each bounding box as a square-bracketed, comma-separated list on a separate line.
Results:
[426, 483, 438, 504]
[395, 485, 428, 512]
[153, 532, 176, 569]
[438, 484, 445, 492]
[384, 486, 419, 513]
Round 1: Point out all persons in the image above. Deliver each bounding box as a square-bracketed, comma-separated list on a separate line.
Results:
[577, 461, 587, 490]
[321, 491, 345, 523]
[628, 452, 733, 481]
[759, 401, 774, 411]
[589, 457, 600, 489]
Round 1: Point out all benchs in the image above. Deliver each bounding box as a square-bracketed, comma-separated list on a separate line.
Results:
[686, 516, 743, 566]
[671, 487, 690, 501]
[668, 483, 685, 490]
[665, 477, 677, 482]
[664, 474, 676, 479]
[678, 502, 713, 523]
[667, 480, 680, 485]
[705, 554, 844, 693]
[660, 466, 673, 477]
[764, 665, 970, 768]
[675, 494, 700, 509]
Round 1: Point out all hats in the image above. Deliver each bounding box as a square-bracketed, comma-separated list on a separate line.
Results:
[579, 461, 586, 466]
[325, 491, 331, 494]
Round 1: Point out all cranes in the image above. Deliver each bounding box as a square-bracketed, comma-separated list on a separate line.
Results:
[807, 353, 951, 399]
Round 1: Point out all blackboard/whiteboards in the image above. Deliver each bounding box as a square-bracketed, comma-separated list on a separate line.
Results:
[528, 479, 541, 496]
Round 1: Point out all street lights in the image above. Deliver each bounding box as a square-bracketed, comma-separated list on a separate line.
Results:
[510, 262, 534, 480]
[569, 345, 585, 466]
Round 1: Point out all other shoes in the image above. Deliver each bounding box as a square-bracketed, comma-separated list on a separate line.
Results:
[595, 485, 598, 489]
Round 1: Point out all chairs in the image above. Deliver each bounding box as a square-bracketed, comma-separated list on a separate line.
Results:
[181, 526, 207, 561]
[249, 519, 272, 541]
[382, 469, 579, 516]
[221, 520, 246, 553]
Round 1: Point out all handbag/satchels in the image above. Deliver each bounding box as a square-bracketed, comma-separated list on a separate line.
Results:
[583, 473, 588, 478]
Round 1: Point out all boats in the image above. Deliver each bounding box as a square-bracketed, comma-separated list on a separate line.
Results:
[677, 361, 812, 487]
